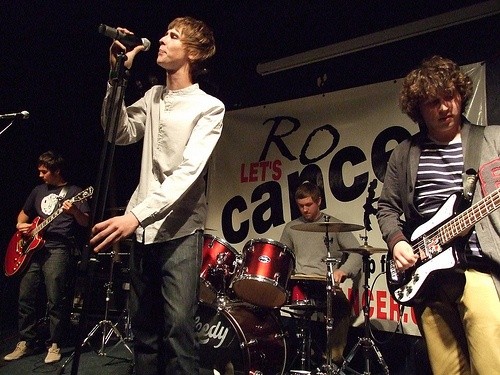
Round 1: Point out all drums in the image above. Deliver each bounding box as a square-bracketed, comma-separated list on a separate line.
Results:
[230, 238, 297, 307]
[199, 233, 240, 304]
[286, 277, 326, 306]
[174, 293, 287, 375]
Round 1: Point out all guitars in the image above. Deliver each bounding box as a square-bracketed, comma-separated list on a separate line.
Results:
[5, 186, 94, 278]
[386, 187, 500, 307]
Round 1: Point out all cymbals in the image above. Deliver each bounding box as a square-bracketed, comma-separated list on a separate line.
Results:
[289, 222, 366, 233]
[337, 244, 390, 257]
[108, 205, 127, 211]
[204, 227, 219, 231]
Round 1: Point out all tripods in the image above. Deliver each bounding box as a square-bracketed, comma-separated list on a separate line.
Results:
[55, 217, 133, 375]
[285, 228, 389, 375]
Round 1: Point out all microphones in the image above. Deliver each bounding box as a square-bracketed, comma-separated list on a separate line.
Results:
[0, 110, 29, 119]
[98, 23, 151, 52]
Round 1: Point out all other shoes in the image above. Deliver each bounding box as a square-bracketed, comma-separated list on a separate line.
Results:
[44, 343, 61, 363]
[4, 341, 30, 361]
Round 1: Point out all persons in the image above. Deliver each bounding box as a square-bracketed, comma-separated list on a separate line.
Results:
[279, 180, 362, 363]
[4, 150, 93, 363]
[91, 17, 225, 375]
[377, 55, 500, 375]
